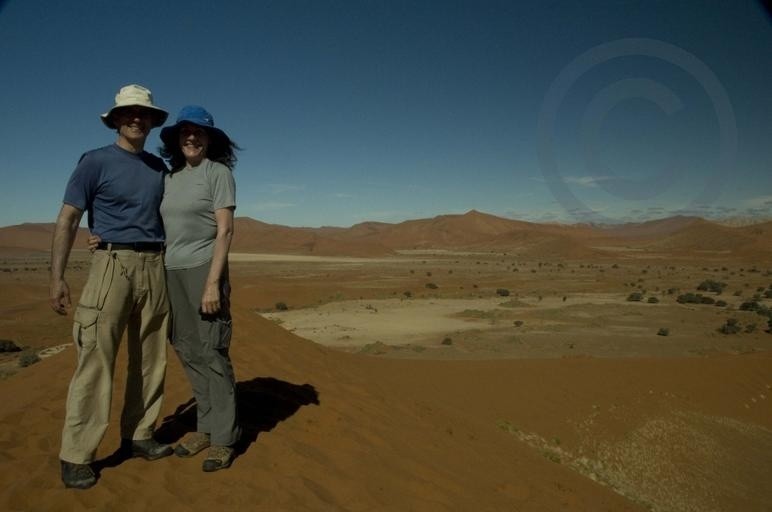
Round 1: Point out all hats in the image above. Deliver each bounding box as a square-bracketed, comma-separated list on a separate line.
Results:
[160, 106, 230, 148]
[101, 85, 169, 130]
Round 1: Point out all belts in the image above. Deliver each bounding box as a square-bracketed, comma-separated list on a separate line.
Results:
[93, 241, 165, 252]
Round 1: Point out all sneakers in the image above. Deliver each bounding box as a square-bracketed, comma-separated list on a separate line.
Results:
[61, 460, 96, 490]
[175, 432, 213, 457]
[121, 440, 171, 461]
[203, 445, 233, 472]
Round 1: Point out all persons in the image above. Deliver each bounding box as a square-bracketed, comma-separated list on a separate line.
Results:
[49, 83, 172, 488]
[86, 104, 240, 473]
